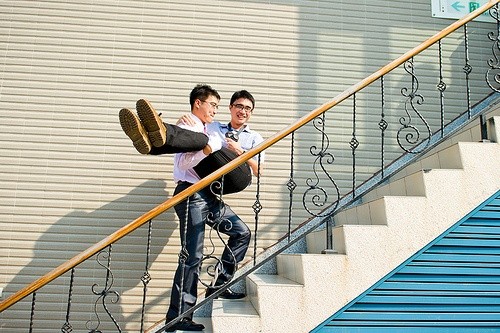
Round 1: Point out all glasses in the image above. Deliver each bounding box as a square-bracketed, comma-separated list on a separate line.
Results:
[200, 98, 220, 111]
[232, 103, 253, 113]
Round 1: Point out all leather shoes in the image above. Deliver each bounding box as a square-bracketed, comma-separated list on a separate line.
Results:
[166, 318, 205, 332]
[204, 284, 247, 299]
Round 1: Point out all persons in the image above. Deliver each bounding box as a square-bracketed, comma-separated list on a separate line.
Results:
[164, 84, 252, 332]
[118, 89, 265, 195]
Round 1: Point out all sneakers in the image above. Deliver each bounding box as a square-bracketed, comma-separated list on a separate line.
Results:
[117, 108, 151, 154]
[136, 100, 166, 149]
